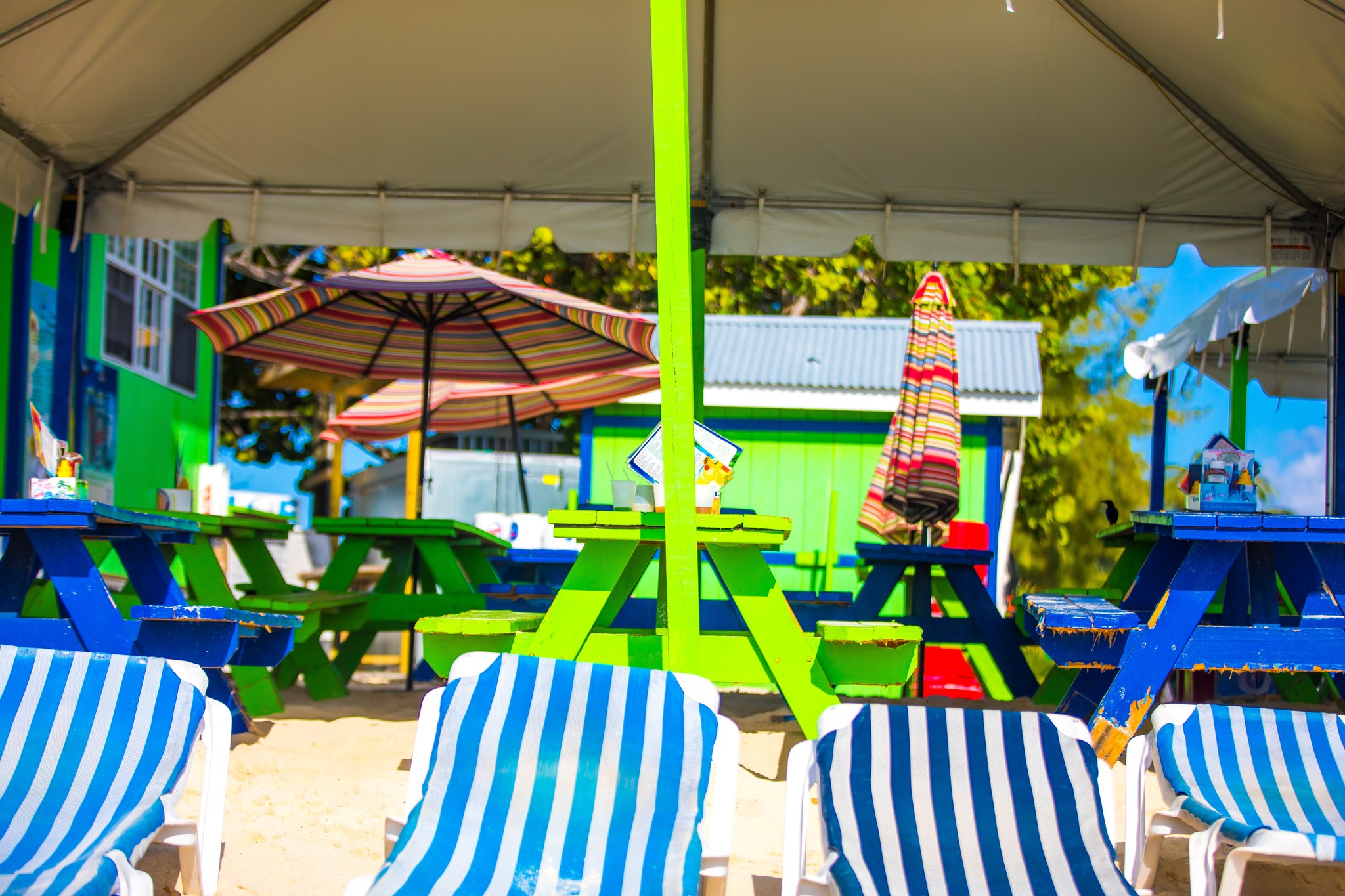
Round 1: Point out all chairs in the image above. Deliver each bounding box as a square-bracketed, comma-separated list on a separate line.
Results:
[1126, 700, 1345, 896]
[0, 643, 233, 896]
[782, 703, 1141, 896]
[346, 650, 739, 896]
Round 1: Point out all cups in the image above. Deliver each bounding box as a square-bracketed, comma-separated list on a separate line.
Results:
[611, 480, 637, 511]
[695, 484, 716, 514]
[474, 512, 577, 550]
[156, 488, 193, 513]
[654, 484, 665, 513]
[631, 485, 655, 512]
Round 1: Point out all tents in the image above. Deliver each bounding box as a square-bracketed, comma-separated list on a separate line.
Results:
[1124, 268, 1344, 516]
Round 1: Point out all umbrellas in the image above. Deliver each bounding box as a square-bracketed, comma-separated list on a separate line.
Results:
[0, 0, 1345, 661]
[884, 273, 959, 622]
[184, 248, 667, 698]
[859, 412, 948, 546]
[318, 360, 661, 511]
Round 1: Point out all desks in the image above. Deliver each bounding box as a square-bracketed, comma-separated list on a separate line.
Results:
[489, 549, 579, 580]
[22, 510, 348, 721]
[277, 518, 513, 688]
[524, 510, 840, 740]
[853, 542, 1039, 698]
[0, 498, 248, 735]
[1061, 510, 1345, 763]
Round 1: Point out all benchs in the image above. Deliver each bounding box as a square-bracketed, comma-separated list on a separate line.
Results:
[132, 603, 297, 674]
[238, 589, 381, 643]
[784, 591, 853, 632]
[414, 607, 541, 680]
[1017, 586, 1138, 669]
[812, 617, 922, 696]
[478, 582, 554, 613]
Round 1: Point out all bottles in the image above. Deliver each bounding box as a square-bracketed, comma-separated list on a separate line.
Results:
[1237, 469, 1254, 485]
[1190, 480, 1199, 495]
[1230, 463, 1239, 484]
[1203, 460, 1230, 484]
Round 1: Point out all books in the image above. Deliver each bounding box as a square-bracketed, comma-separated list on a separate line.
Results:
[1177, 432, 1261, 495]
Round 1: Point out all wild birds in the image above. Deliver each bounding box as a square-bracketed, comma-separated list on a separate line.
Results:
[1100, 501, 1119, 527]
